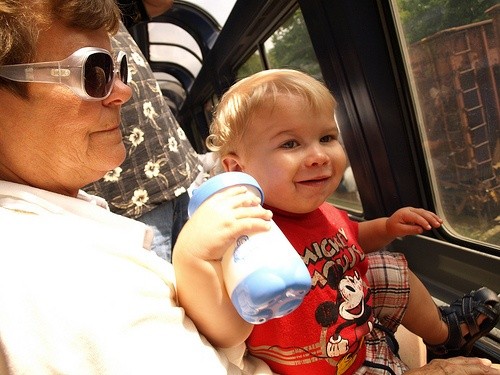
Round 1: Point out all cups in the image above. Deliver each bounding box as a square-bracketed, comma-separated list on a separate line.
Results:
[188, 171, 312, 324]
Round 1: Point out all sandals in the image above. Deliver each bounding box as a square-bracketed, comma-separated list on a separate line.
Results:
[423, 286, 500, 356]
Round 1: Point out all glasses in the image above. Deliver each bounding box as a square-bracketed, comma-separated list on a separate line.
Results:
[0, 46, 129, 101]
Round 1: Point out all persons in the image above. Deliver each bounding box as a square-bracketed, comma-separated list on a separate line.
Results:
[171, 69, 500, 375]
[0, 0, 500, 375]
[80, 18, 202, 263]
